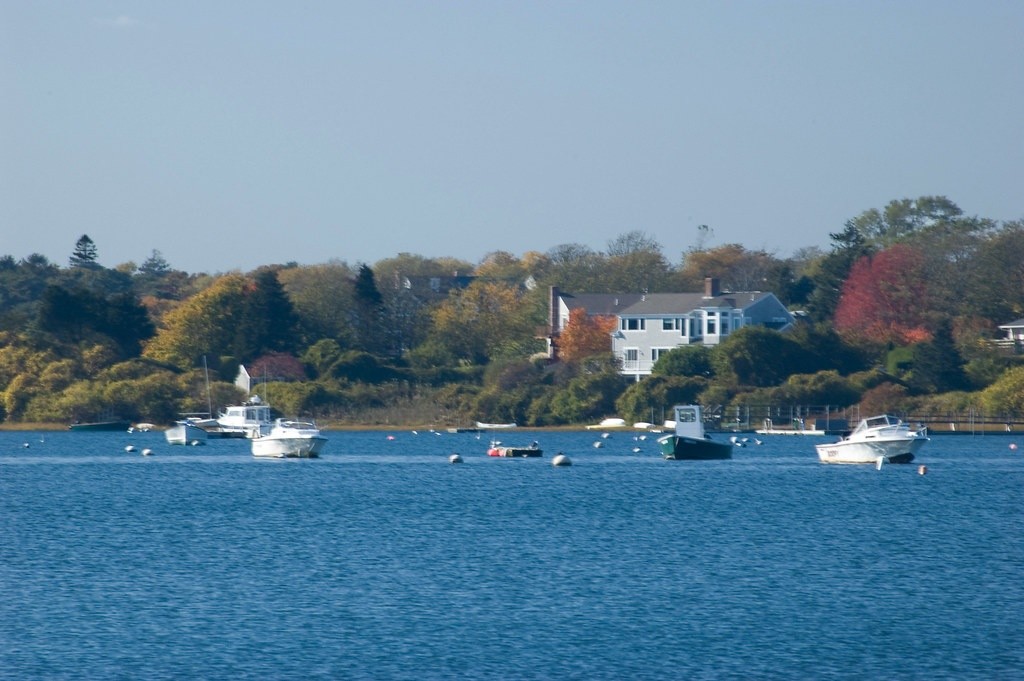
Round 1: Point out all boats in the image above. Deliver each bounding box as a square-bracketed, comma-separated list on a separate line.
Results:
[815, 414, 931, 471]
[164, 419, 209, 446]
[656, 404, 734, 461]
[251, 417, 329, 458]
[216, 395, 271, 438]
[487, 440, 544, 457]
[172, 416, 217, 427]
